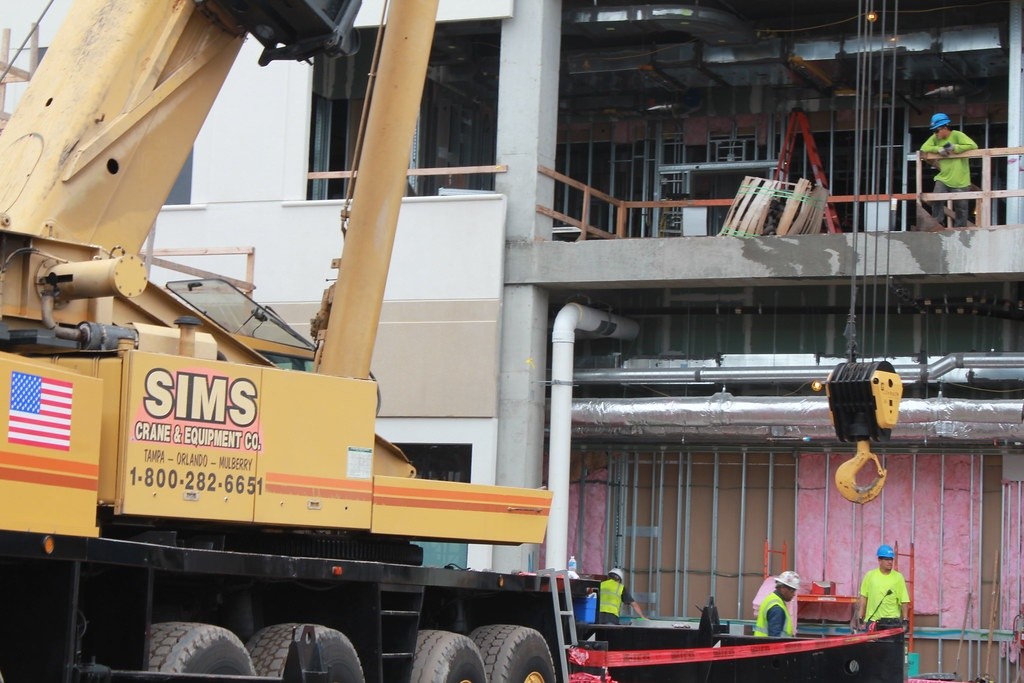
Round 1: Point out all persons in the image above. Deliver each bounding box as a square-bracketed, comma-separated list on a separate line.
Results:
[752, 571, 802, 639]
[594, 568, 651, 625]
[851, 545, 910, 638]
[918, 113, 979, 231]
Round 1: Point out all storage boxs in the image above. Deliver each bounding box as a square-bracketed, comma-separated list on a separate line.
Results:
[573, 592, 598, 624]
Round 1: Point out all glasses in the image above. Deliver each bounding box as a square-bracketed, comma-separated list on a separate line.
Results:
[934, 128, 944, 134]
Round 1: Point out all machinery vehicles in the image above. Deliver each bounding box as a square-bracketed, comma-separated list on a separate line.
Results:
[0, 2, 914, 682]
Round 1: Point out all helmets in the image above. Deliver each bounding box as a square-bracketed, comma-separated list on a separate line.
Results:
[877, 545, 896, 558]
[774, 571, 801, 590]
[608, 568, 622, 582]
[929, 113, 952, 130]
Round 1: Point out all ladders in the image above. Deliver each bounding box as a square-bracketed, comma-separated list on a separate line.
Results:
[766, 105, 840, 231]
